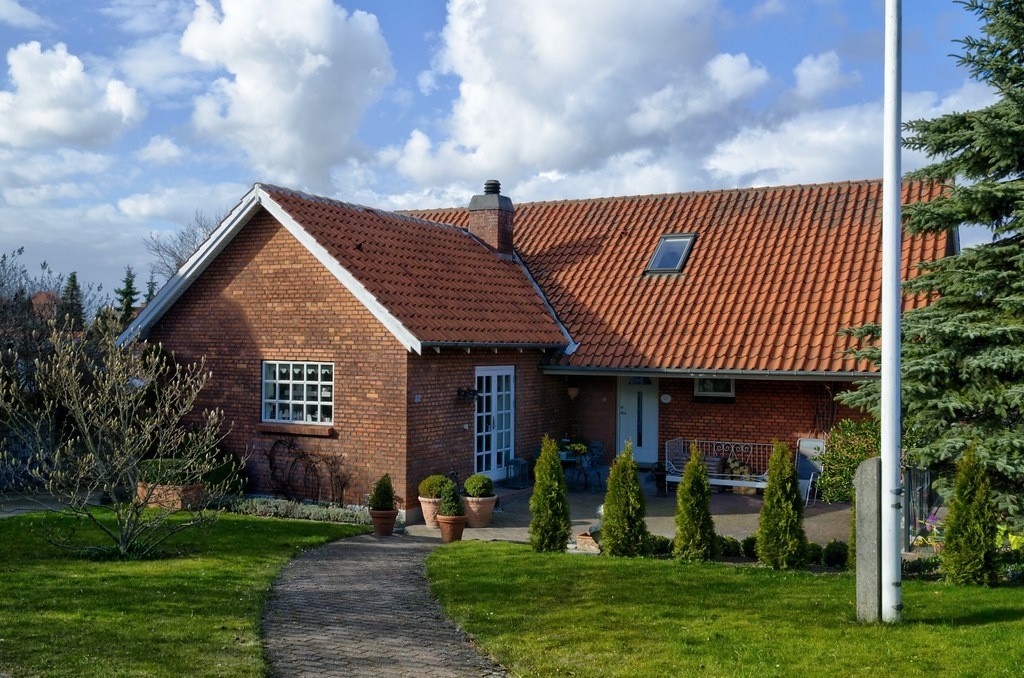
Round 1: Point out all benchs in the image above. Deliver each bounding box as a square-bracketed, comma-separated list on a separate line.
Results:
[664, 439, 778, 493]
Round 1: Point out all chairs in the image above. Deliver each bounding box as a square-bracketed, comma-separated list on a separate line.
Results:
[794, 439, 826, 508]
[574, 440, 608, 495]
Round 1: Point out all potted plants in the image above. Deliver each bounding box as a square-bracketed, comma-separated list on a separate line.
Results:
[135, 457, 202, 512]
[436, 479, 468, 544]
[418, 475, 448, 529]
[459, 473, 498, 528]
[368, 472, 399, 538]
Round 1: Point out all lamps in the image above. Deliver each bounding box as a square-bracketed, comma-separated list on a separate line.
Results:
[567, 387, 581, 400]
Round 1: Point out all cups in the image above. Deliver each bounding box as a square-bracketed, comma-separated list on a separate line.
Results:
[559, 451, 567, 459]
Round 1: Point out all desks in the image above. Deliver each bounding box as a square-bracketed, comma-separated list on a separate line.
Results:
[557, 456, 584, 472]
